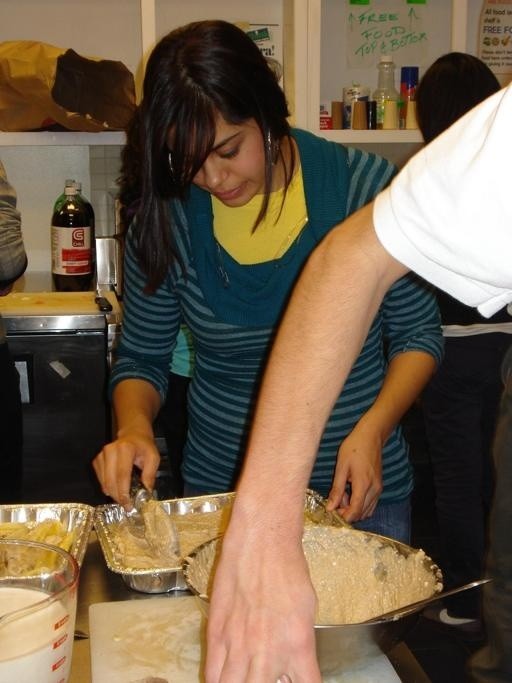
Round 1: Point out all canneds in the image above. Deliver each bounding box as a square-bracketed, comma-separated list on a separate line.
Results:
[343, 87, 371, 129]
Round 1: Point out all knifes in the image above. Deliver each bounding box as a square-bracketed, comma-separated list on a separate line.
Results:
[95, 289, 113, 311]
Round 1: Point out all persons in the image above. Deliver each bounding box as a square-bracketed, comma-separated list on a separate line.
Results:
[200, 81, 512, 683]
[393, 50, 512, 683]
[0, 159, 29, 506]
[90, 19, 449, 546]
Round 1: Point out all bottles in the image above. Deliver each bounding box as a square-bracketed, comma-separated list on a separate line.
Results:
[372, 55, 419, 129]
[50, 178, 96, 292]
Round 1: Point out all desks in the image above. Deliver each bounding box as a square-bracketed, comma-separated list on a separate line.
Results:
[69, 526, 434, 683]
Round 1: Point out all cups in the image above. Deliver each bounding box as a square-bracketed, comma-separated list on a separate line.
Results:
[0, 539, 80, 683]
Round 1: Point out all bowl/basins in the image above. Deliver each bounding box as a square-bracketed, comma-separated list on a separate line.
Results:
[182, 524, 444, 677]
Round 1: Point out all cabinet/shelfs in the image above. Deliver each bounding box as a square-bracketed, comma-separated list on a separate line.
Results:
[0, 0, 511, 337]
